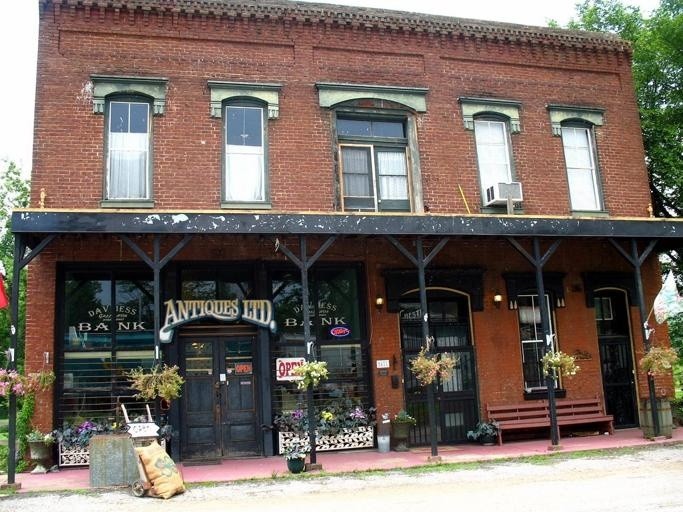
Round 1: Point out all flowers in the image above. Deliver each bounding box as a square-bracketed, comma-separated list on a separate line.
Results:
[0, 368, 39, 397]
[407, 346, 461, 386]
[391, 409, 417, 427]
[58, 414, 172, 445]
[541, 350, 581, 379]
[639, 346, 680, 376]
[274, 404, 378, 435]
[295, 360, 328, 391]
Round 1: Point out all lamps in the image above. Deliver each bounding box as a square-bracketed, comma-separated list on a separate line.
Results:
[375, 292, 384, 315]
[494, 288, 503, 310]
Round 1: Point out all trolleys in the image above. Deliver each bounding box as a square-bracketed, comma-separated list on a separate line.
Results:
[120, 403, 162, 498]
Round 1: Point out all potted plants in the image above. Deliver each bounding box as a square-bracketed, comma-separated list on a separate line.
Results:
[286, 449, 309, 473]
[26, 428, 54, 474]
[466, 422, 500, 445]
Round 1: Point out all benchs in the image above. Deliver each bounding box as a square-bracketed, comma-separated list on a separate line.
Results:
[488, 393, 615, 445]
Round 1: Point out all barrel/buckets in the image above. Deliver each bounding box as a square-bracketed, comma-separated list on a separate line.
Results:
[640, 396, 672, 441]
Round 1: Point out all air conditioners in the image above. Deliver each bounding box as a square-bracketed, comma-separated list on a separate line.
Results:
[486, 181, 524, 207]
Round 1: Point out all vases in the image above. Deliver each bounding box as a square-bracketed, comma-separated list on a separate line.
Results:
[393, 423, 411, 452]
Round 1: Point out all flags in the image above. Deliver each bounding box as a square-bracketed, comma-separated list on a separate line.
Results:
[653, 270, 682, 324]
